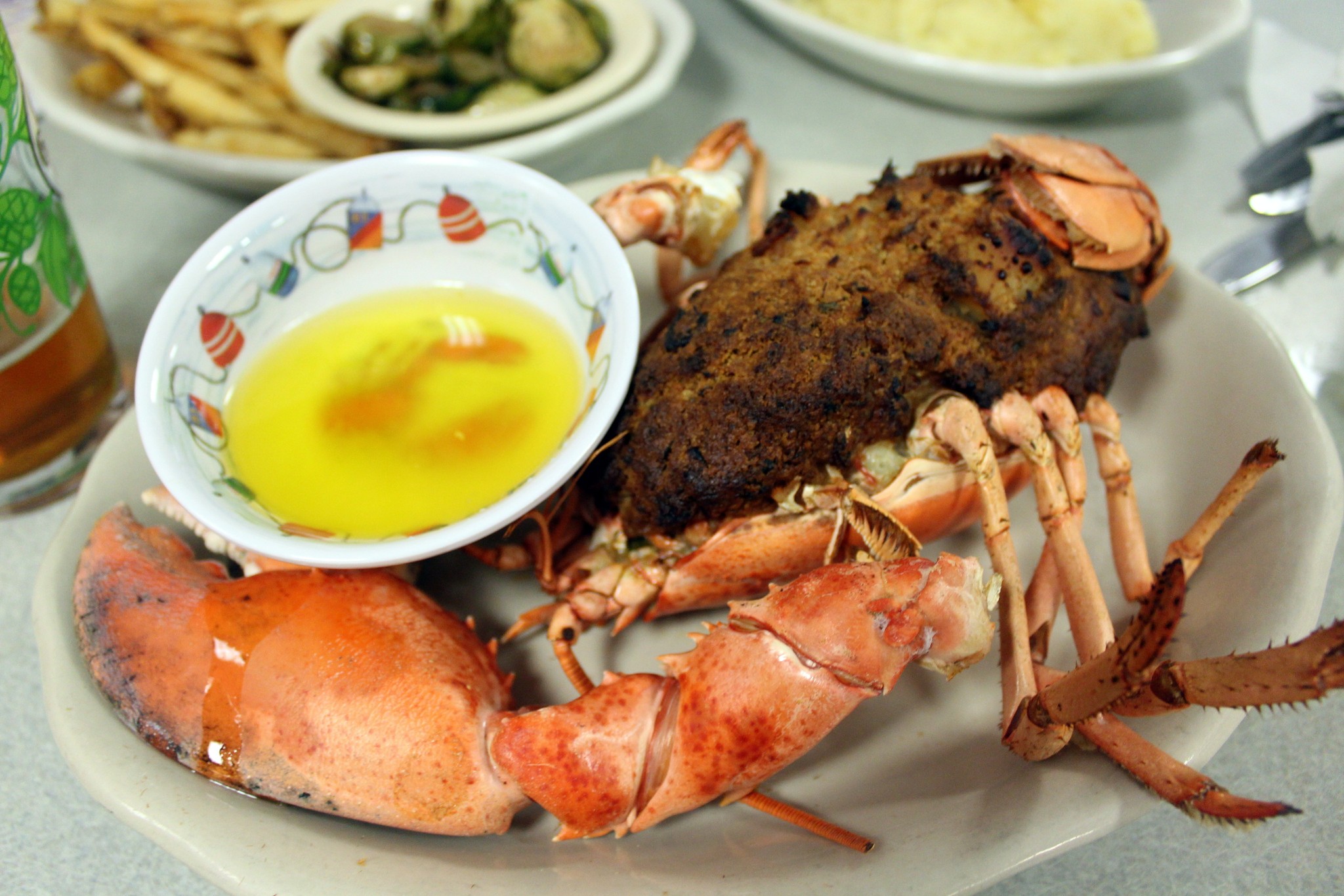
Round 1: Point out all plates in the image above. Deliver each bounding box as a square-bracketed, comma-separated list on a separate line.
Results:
[35, 161, 1344, 896]
[0, 0, 698, 196]
[736, 0, 1251, 117]
[284, 0, 659, 143]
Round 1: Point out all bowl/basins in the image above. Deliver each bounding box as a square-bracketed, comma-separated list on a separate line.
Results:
[133, 148, 642, 571]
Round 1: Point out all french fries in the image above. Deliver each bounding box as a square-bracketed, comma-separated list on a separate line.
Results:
[35, 0, 390, 163]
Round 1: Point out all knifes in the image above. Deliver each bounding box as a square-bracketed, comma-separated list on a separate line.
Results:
[1197, 210, 1335, 296]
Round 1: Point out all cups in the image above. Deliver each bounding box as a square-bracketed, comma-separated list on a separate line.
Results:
[0, 19, 134, 517]
